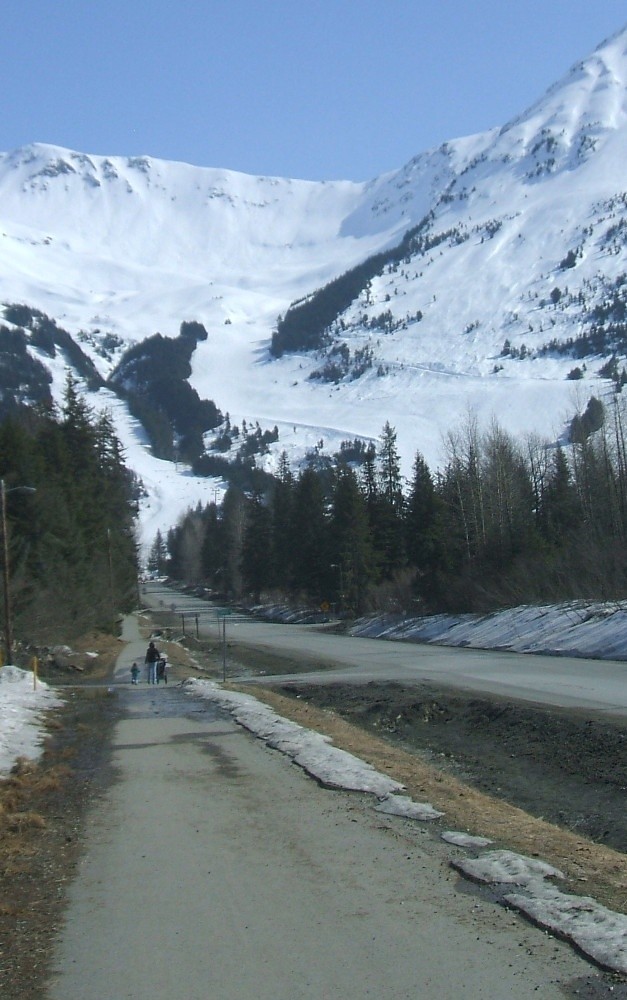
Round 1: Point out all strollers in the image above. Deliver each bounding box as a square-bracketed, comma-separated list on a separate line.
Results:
[156, 658, 167, 684]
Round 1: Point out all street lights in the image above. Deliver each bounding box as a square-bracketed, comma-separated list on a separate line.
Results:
[330, 563, 344, 624]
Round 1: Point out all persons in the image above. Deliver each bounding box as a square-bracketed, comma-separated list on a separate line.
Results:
[130, 662, 141, 684]
[145, 642, 160, 685]
